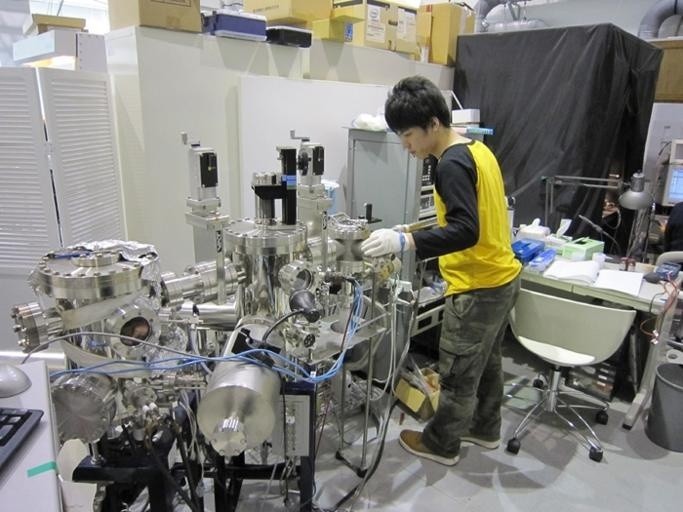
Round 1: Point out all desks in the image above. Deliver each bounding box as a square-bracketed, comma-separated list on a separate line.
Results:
[521, 252, 683, 430]
[0, 359, 68, 512]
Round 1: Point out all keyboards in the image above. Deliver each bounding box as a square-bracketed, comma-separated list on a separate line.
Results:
[0, 407, 44, 471]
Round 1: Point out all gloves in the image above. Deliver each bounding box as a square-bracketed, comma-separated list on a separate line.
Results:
[360, 229, 410, 258]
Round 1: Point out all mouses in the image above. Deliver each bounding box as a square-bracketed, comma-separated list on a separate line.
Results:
[0, 362, 32, 398]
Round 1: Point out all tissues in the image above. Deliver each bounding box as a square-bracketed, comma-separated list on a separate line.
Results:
[518, 218, 550, 239]
[545, 219, 572, 255]
[562, 235, 606, 261]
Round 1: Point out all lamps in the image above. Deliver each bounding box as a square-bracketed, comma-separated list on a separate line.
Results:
[544, 169, 654, 227]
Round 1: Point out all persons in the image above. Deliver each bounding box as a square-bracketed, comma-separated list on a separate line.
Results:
[663, 201, 683, 273]
[360, 75, 526, 468]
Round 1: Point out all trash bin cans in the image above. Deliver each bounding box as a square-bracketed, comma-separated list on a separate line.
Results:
[644, 363, 683, 453]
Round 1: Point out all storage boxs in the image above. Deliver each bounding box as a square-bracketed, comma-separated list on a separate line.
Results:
[243, 0, 476, 66]
[394, 367, 441, 419]
[108, 0, 201, 33]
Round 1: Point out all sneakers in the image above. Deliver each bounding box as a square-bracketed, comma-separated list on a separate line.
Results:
[399, 430, 460, 466]
[459, 425, 501, 449]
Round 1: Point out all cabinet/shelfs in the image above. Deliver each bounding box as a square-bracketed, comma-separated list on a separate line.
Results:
[345, 129, 484, 396]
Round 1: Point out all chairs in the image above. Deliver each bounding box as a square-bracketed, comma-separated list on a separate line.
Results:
[640, 202, 683, 254]
[507, 287, 638, 462]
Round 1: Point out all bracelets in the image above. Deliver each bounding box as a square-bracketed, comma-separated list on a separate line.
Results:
[399, 232, 407, 253]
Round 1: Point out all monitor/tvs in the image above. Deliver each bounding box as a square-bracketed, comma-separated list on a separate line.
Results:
[662, 164, 683, 207]
